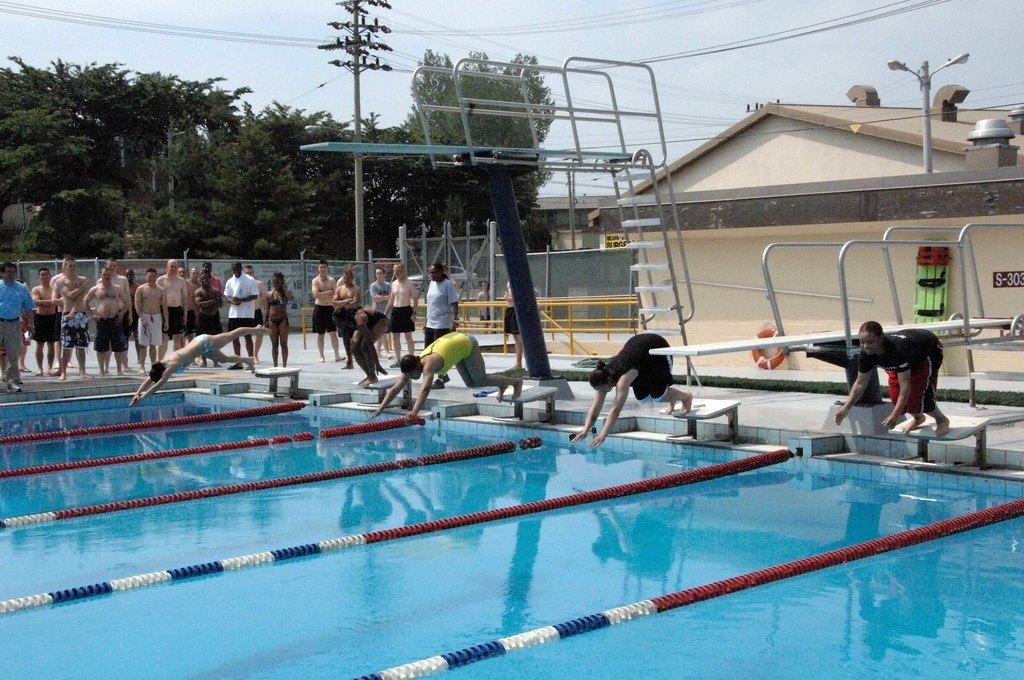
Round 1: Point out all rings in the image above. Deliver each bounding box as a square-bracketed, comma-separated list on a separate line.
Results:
[595, 441, 598, 444]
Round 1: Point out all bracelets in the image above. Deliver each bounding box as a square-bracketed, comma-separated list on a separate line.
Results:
[413, 312, 416, 314]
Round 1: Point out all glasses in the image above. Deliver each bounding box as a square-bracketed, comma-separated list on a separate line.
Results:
[104, 274, 110, 276]
[69, 264, 75, 267]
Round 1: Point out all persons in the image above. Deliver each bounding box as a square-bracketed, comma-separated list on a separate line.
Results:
[570, 334, 693, 447]
[834, 321, 950, 437]
[129, 324, 272, 406]
[0, 257, 540, 394]
[366, 332, 523, 426]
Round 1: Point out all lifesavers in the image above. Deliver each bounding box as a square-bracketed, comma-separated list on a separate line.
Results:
[751, 326, 786, 370]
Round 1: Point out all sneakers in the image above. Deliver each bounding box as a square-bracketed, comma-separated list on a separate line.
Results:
[7, 380, 22, 392]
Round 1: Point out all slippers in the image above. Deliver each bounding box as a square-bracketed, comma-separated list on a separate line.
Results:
[20, 366, 30, 372]
[227, 365, 243, 369]
